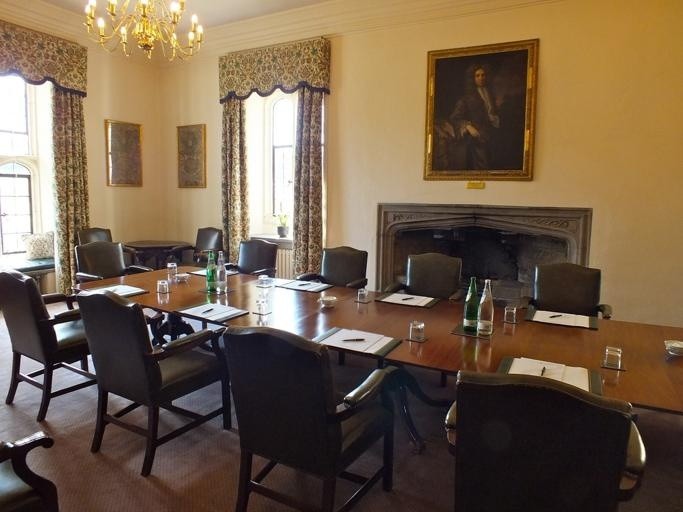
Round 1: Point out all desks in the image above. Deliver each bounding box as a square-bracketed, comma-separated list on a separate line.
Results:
[125, 238, 191, 269]
[67, 265, 683, 417]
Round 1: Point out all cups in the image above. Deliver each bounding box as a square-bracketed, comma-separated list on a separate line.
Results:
[320, 296, 337, 307]
[602, 370, 621, 390]
[409, 342, 423, 358]
[157, 262, 273, 315]
[358, 289, 369, 303]
[505, 324, 516, 340]
[505, 306, 516, 322]
[604, 346, 622, 367]
[358, 304, 370, 315]
[407, 319, 424, 340]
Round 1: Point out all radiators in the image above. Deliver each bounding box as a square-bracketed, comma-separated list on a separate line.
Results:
[251, 237, 293, 279]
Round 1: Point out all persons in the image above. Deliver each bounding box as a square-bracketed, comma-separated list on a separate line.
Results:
[448, 65, 505, 171]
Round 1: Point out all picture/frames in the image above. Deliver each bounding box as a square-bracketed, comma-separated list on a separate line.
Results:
[423, 38, 540, 181]
[105, 119, 143, 187]
[176, 123, 207, 188]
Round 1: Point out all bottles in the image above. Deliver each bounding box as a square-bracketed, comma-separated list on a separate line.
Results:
[463, 275, 493, 335]
[461, 335, 492, 372]
[205, 249, 226, 296]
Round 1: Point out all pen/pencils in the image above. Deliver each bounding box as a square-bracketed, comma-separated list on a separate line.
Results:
[402, 297, 414, 300]
[203, 308, 213, 313]
[550, 315, 562, 318]
[541, 367, 545, 376]
[299, 283, 311, 286]
[342, 339, 365, 341]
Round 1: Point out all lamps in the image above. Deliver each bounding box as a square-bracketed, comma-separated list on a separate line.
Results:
[81, 0, 205, 65]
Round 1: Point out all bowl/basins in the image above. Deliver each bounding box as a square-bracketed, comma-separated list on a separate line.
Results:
[665, 338, 682, 356]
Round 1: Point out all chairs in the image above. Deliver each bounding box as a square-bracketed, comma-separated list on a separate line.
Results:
[1, 269, 97, 422]
[441, 370, 647, 512]
[518, 261, 613, 320]
[375, 252, 463, 388]
[192, 227, 223, 268]
[76, 289, 230, 477]
[1, 429, 58, 511]
[76, 227, 113, 247]
[222, 324, 400, 512]
[75, 241, 152, 284]
[295, 246, 367, 366]
[225, 239, 278, 278]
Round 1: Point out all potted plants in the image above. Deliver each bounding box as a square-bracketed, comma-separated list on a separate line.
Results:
[272, 212, 289, 237]
